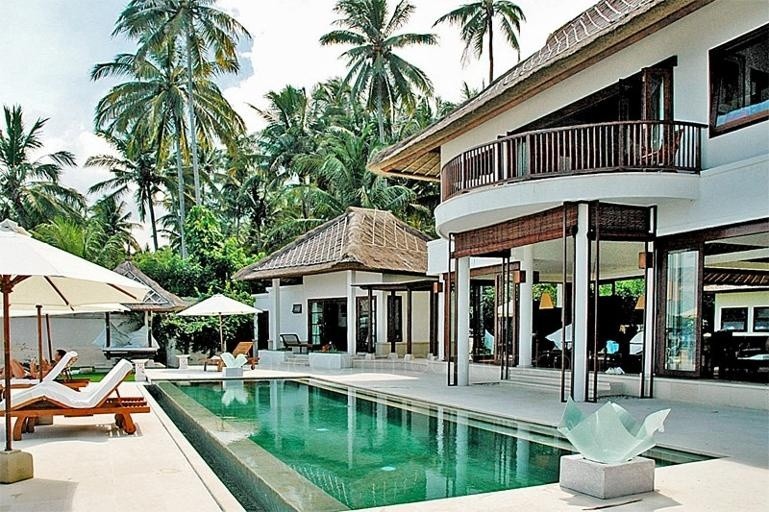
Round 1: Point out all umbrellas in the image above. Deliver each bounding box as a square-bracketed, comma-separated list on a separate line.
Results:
[0, 217, 147, 452]
[176, 292, 263, 354]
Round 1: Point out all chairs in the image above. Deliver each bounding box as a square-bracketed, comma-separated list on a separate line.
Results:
[204, 342, 260, 372]
[0, 350, 150, 441]
[280, 334, 313, 354]
